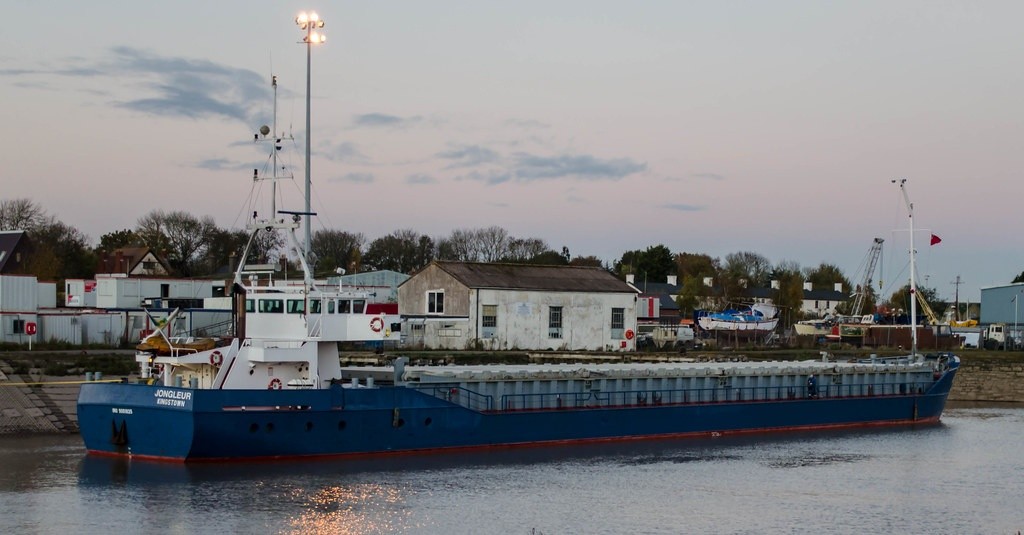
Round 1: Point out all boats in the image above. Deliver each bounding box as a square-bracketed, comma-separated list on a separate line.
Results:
[76, 62, 961, 473]
[698, 308, 781, 333]
[794, 311, 875, 337]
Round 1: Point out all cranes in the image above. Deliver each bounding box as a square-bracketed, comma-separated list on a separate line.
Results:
[842, 236, 883, 338]
[914, 283, 940, 327]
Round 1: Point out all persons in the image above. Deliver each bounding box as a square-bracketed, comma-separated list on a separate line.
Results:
[152, 374, 163, 386]
[807, 374, 817, 396]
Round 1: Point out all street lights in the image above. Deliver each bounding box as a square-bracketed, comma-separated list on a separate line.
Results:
[294, 12, 329, 252]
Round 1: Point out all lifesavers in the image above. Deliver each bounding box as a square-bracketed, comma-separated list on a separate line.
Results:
[209, 350, 224, 368]
[369, 317, 385, 332]
[269, 378, 283, 390]
